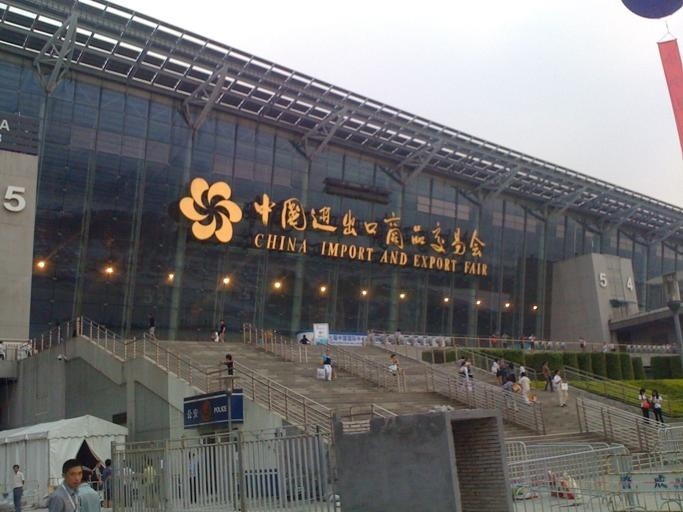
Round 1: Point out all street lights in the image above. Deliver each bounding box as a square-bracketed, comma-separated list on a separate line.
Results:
[210, 375, 249, 512]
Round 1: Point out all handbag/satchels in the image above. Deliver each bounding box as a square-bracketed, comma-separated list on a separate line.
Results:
[561, 381, 568, 391]
[91, 470, 99, 481]
[387, 365, 397, 373]
[491, 366, 498, 375]
[643, 400, 655, 409]
[511, 383, 523, 393]
[528, 393, 537, 402]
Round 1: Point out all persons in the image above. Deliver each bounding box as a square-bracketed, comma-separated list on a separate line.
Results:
[520, 334, 616, 352]
[216, 353, 235, 389]
[457, 356, 473, 385]
[12, 465, 25, 512]
[320, 349, 336, 381]
[219, 319, 227, 343]
[0, 340, 5, 360]
[47, 450, 196, 512]
[386, 354, 398, 376]
[649, 389, 665, 430]
[638, 388, 650, 424]
[492, 359, 569, 408]
[148, 313, 156, 341]
[300, 335, 311, 344]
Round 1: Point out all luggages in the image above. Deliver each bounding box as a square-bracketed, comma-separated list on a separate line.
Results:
[317, 368, 326, 380]
